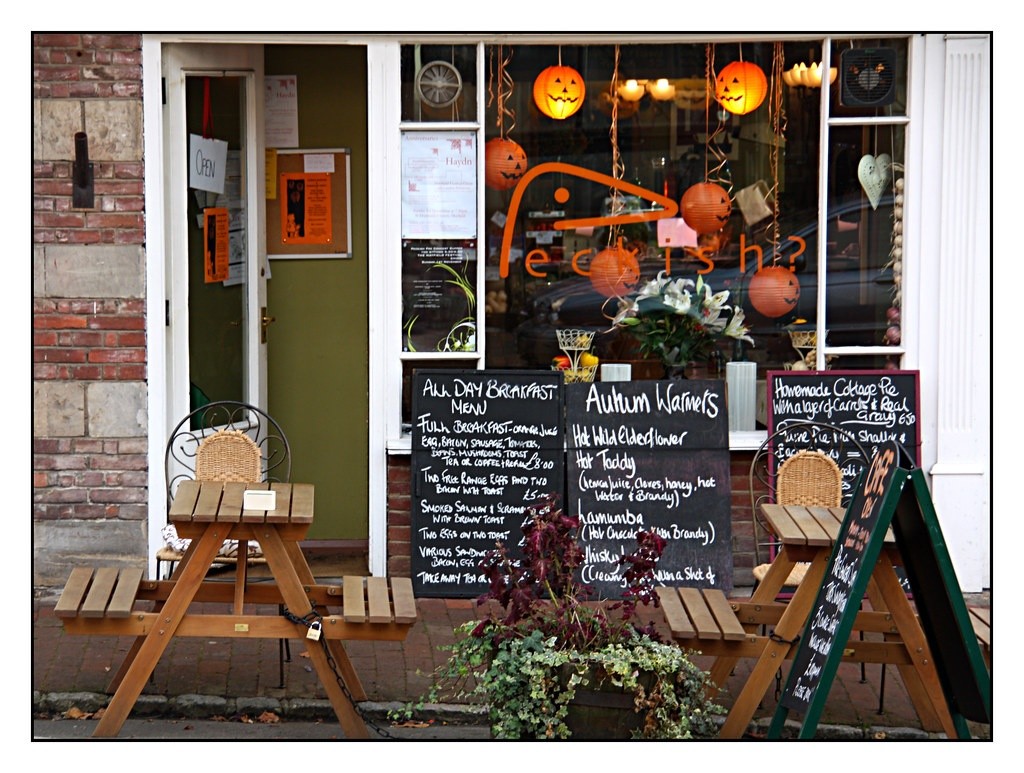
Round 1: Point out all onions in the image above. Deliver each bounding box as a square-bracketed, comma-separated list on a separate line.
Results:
[884, 308, 902, 371]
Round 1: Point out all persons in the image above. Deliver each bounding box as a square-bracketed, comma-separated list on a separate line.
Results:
[698, 225, 731, 257]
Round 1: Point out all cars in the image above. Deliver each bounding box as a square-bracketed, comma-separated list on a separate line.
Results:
[512, 193, 894, 370]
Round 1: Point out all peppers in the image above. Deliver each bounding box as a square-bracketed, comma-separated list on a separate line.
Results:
[565, 346, 599, 382]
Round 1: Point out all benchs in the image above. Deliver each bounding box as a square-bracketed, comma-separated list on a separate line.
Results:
[655, 575, 744, 641]
[56, 564, 146, 623]
[338, 573, 417, 642]
[967, 603, 990, 659]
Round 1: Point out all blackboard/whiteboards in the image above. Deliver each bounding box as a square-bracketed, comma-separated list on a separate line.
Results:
[567, 380, 733, 602]
[788, 439, 902, 707]
[415, 372, 563, 596]
[770, 374, 920, 596]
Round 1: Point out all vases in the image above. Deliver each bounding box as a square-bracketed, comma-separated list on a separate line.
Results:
[485, 650, 680, 739]
[664, 349, 694, 382]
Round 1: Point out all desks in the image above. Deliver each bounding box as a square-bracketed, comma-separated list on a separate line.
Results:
[655, 504, 957, 741]
[56, 484, 426, 738]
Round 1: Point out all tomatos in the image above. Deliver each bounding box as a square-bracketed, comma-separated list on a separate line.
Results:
[551, 356, 571, 370]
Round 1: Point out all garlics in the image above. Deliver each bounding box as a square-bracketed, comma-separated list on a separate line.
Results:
[891, 177, 904, 301]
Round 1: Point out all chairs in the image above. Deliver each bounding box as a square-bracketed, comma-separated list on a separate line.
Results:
[750, 421, 872, 683]
[156, 400, 293, 691]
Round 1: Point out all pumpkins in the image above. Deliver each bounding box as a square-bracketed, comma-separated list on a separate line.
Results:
[789, 318, 832, 372]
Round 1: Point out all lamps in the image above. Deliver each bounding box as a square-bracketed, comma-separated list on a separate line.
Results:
[533, 46, 587, 119]
[617, 77, 676, 102]
[716, 46, 767, 116]
[781, 46, 838, 103]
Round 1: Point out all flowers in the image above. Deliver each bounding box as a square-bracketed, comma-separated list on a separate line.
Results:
[604, 271, 758, 365]
[395, 500, 728, 741]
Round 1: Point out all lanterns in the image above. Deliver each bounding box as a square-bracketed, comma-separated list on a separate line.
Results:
[590, 246, 641, 299]
[485, 137, 528, 191]
[715, 62, 768, 116]
[598, 79, 714, 118]
[533, 64, 585, 120]
[749, 267, 802, 318]
[680, 182, 732, 234]
